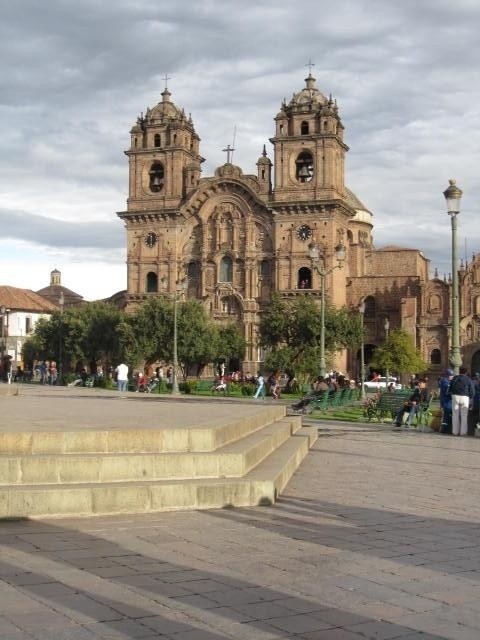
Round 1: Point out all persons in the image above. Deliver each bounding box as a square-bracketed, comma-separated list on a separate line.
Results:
[1, 354, 57, 385]
[394, 373, 429, 427]
[438, 367, 479, 436]
[314, 371, 361, 395]
[388, 383, 395, 392]
[79, 358, 133, 393]
[370, 371, 381, 382]
[134, 366, 173, 393]
[224, 370, 280, 401]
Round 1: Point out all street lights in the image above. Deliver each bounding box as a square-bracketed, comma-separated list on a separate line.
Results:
[306, 241, 346, 379]
[55, 291, 67, 384]
[358, 297, 367, 400]
[0, 305, 6, 363]
[445, 178, 464, 377]
[161, 275, 190, 394]
[384, 318, 391, 388]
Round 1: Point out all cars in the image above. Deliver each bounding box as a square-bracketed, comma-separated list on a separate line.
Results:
[358, 376, 398, 389]
[263, 372, 297, 388]
[215, 371, 247, 383]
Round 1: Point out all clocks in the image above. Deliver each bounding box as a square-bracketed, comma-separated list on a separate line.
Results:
[296, 223, 312, 241]
[145, 229, 159, 248]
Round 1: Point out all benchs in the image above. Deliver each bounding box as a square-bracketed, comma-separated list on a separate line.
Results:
[365, 392, 433, 429]
[126, 377, 165, 393]
[84, 376, 94, 387]
[194, 379, 231, 396]
[301, 383, 313, 398]
[308, 388, 361, 411]
